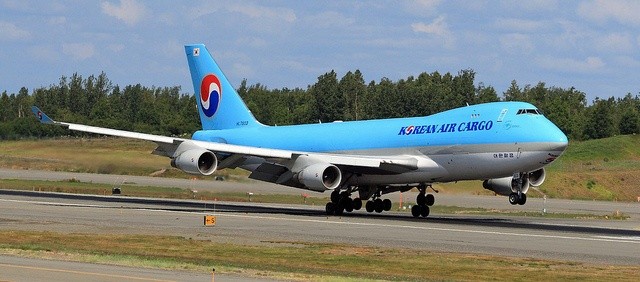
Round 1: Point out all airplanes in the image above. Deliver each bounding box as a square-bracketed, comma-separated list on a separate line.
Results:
[31, 44, 568, 217]
